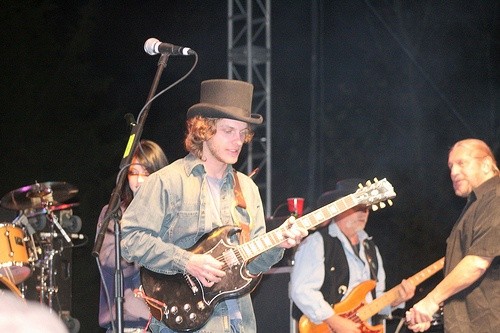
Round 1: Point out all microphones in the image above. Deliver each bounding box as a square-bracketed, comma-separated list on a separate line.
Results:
[144, 37, 196, 56]
[54, 220, 75, 247]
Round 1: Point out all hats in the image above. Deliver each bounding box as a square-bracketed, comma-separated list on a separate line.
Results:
[316, 178, 366, 205]
[186, 79, 263, 125]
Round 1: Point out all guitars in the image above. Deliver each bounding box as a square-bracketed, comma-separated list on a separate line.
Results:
[139, 176, 397, 333]
[297, 256, 446, 333]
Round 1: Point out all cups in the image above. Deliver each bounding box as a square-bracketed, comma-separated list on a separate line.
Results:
[287, 198, 305, 218]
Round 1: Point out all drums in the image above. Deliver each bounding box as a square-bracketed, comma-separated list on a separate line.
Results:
[0, 222, 34, 286]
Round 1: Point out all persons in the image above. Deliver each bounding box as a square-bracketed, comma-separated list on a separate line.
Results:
[122, 80, 307, 333]
[99, 139, 169, 333]
[405, 139, 500, 333]
[287, 178, 415, 333]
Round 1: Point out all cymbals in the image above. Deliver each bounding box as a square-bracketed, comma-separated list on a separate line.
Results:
[0, 181, 79, 210]
[25, 202, 79, 216]
